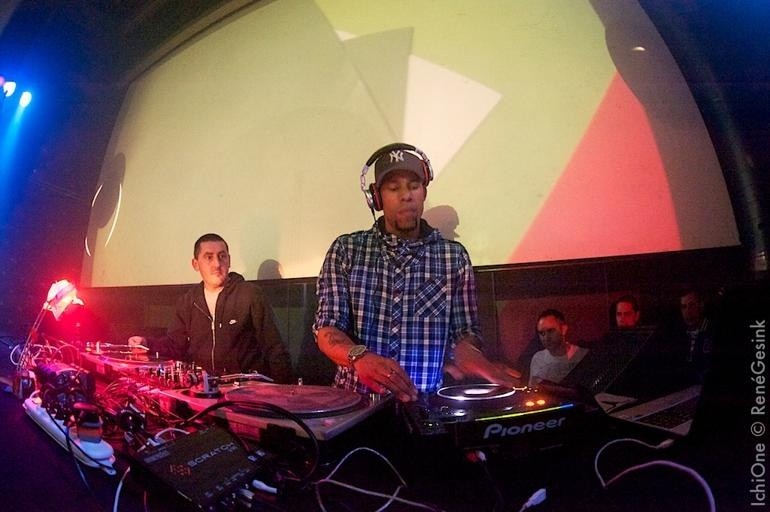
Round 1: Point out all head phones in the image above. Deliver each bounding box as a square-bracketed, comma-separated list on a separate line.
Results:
[360, 143, 434, 212]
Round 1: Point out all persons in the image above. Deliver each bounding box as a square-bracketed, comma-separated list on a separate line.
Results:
[613, 295, 641, 328]
[124, 232, 294, 385]
[527, 308, 589, 387]
[307, 142, 521, 410]
[675, 286, 711, 368]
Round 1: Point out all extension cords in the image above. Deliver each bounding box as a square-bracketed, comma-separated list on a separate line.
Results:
[21, 396, 117, 475]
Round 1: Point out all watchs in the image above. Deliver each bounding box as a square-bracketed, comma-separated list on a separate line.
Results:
[345, 344, 369, 367]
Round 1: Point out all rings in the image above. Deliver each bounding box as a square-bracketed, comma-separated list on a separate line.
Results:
[387, 370, 395, 378]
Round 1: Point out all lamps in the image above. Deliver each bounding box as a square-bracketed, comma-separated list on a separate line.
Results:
[13, 277, 85, 395]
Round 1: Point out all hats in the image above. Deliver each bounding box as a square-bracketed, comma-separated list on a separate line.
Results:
[374, 150, 426, 190]
